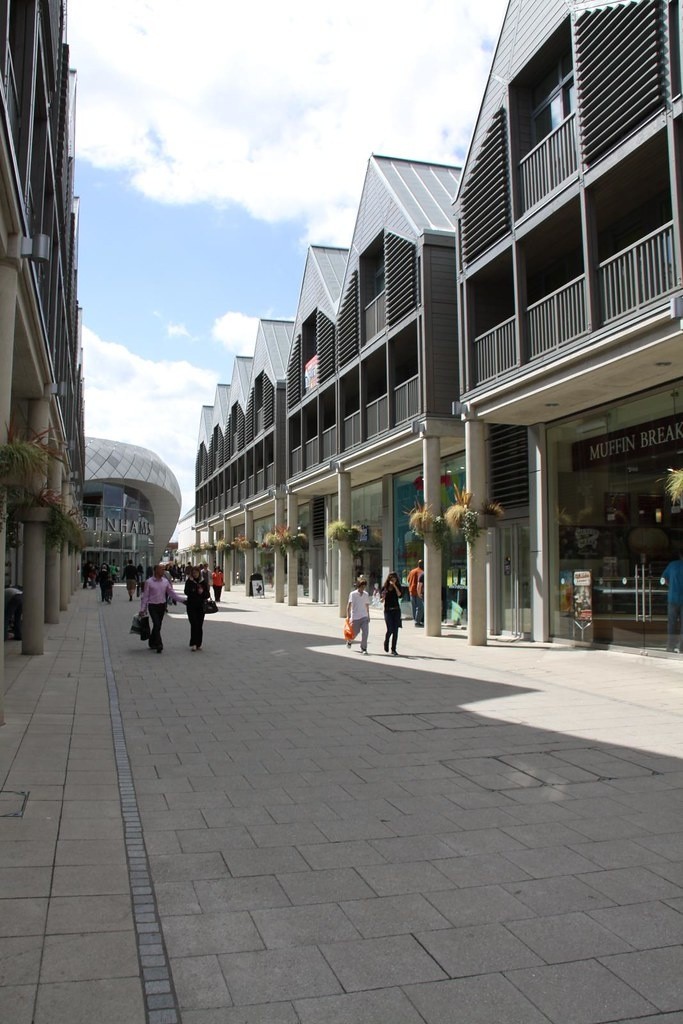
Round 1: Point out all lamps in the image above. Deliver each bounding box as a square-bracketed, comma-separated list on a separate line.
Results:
[191, 526, 195, 530]
[411, 421, 425, 434]
[452, 402, 466, 416]
[268, 490, 274, 497]
[203, 521, 208, 526]
[219, 513, 224, 520]
[240, 504, 245, 510]
[280, 483, 288, 492]
[330, 460, 338, 470]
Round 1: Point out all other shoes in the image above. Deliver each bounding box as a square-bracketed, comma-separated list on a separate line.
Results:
[346, 640, 352, 648]
[362, 647, 367, 654]
[192, 645, 202, 651]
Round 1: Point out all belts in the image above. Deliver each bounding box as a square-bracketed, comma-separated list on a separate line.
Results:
[151, 604, 167, 608]
[385, 607, 398, 610]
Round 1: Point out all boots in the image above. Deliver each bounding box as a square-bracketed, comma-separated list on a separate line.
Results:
[392, 639, 397, 655]
[384, 634, 390, 651]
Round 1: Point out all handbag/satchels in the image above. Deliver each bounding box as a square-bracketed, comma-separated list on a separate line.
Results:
[398, 618, 402, 627]
[167, 595, 176, 606]
[128, 612, 149, 634]
[109, 573, 114, 586]
[345, 618, 354, 640]
[203, 596, 218, 613]
[141, 616, 150, 640]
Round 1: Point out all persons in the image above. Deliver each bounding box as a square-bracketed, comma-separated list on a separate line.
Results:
[121, 560, 139, 601]
[166, 562, 192, 581]
[136, 563, 143, 581]
[139, 564, 187, 653]
[212, 566, 224, 602]
[111, 563, 118, 580]
[159, 563, 172, 612]
[145, 566, 153, 579]
[184, 566, 210, 652]
[95, 564, 114, 604]
[380, 571, 404, 656]
[661, 550, 683, 653]
[407, 559, 424, 626]
[201, 564, 211, 584]
[82, 561, 97, 589]
[345, 577, 370, 654]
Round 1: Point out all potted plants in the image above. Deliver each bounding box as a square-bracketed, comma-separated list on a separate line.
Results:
[0, 421, 91, 555]
[185, 483, 506, 561]
